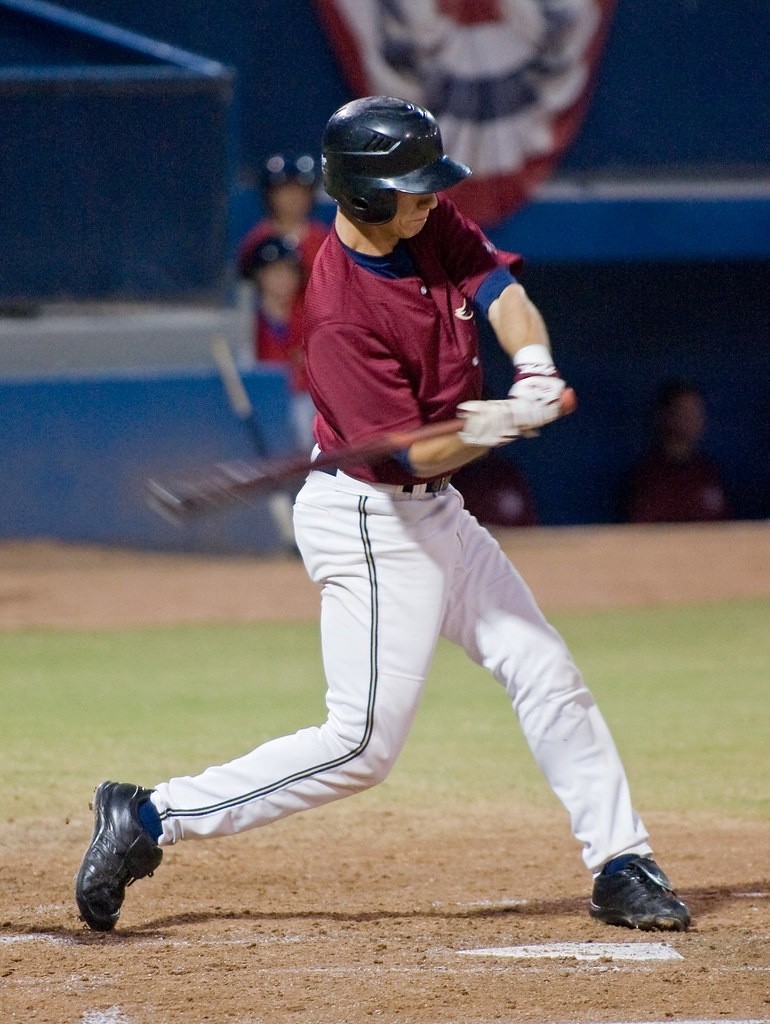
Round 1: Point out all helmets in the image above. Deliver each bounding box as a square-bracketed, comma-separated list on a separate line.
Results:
[319, 95, 474, 224]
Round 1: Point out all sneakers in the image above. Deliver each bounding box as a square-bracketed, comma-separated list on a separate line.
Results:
[74, 780, 163, 931]
[588, 855, 692, 934]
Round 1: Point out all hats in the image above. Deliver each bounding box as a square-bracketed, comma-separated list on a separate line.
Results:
[241, 237, 291, 281]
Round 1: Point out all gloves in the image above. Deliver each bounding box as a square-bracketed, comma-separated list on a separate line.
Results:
[455, 397, 528, 449]
[508, 346, 565, 425]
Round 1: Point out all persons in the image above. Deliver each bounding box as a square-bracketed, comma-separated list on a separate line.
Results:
[77, 97, 690, 933]
[613, 381, 740, 524]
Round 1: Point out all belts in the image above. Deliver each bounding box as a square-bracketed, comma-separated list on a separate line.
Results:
[313, 452, 453, 495]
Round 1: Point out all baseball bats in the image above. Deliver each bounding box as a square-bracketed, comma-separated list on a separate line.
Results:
[135, 389, 580, 527]
